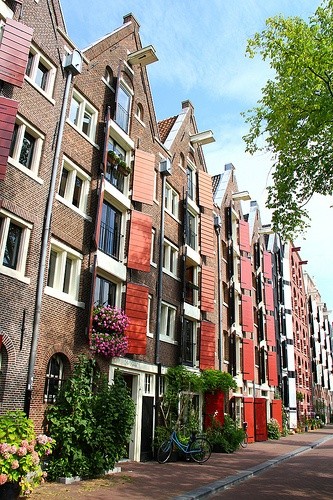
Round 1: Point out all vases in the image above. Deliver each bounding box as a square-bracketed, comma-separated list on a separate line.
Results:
[0, 481, 20, 500]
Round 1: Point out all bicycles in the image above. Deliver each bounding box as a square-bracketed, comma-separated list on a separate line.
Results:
[157, 427, 212, 463]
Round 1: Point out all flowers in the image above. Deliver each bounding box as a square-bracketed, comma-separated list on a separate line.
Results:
[85, 304, 129, 359]
[0, 409, 56, 495]
